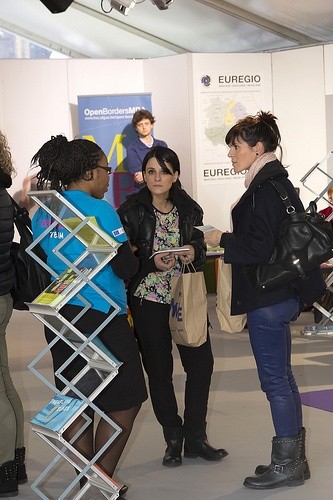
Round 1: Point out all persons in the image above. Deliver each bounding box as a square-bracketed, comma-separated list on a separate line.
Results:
[0, 131, 28, 498]
[123, 109, 168, 194]
[204, 110, 333, 490]
[29, 133, 149, 495]
[116, 146, 229, 466]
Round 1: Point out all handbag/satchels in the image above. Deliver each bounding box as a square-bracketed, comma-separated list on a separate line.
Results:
[216, 256, 247, 333]
[249, 175, 333, 292]
[6, 192, 51, 311]
[168, 255, 209, 347]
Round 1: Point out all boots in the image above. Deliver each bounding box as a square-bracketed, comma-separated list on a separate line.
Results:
[242, 432, 304, 490]
[255, 428, 311, 480]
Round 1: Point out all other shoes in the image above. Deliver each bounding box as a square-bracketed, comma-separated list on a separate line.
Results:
[0, 460, 20, 497]
[13, 446, 30, 484]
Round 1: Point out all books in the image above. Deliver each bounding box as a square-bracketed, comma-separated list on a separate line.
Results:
[30, 216, 121, 490]
[149, 248, 190, 260]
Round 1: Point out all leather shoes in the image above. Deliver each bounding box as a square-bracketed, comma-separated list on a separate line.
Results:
[184, 436, 228, 462]
[162, 439, 183, 467]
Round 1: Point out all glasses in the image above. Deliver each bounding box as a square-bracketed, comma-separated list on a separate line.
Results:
[94, 165, 112, 174]
